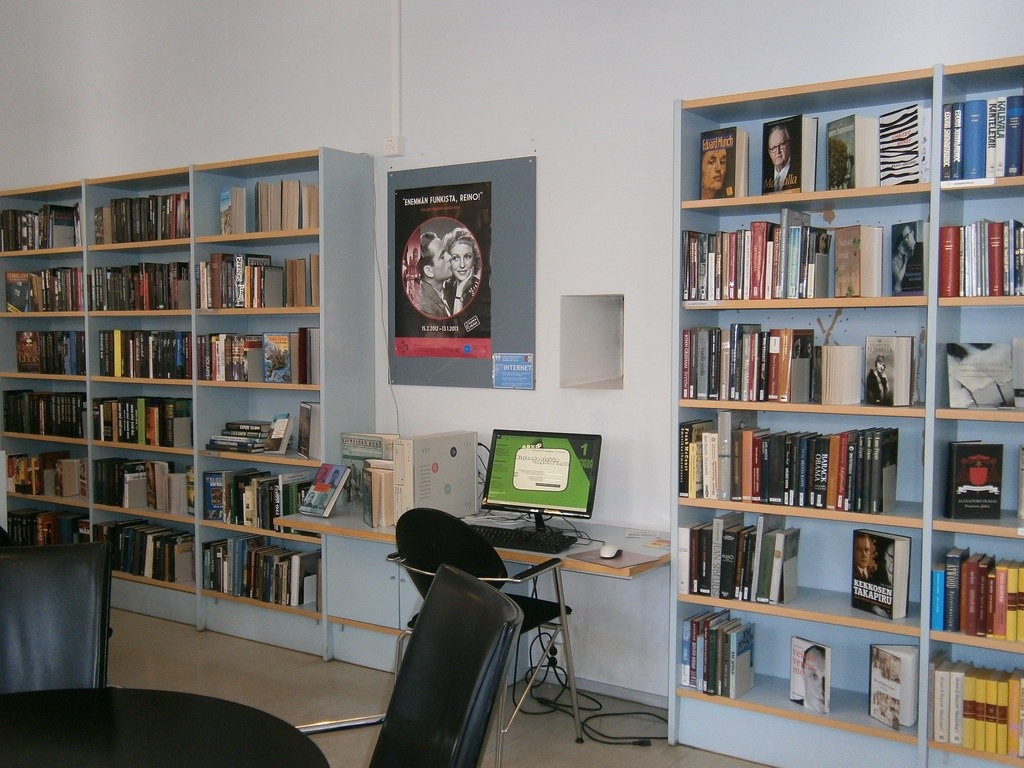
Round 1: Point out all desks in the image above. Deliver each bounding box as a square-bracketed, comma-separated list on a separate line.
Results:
[0, 689, 330, 768]
[273, 499, 671, 674]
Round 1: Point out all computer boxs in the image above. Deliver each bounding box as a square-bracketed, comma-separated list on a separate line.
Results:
[393, 430, 480, 522]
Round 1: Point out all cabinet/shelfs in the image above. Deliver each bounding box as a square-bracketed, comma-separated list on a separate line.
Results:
[0, 147, 375, 662]
[667, 56, 1024, 768]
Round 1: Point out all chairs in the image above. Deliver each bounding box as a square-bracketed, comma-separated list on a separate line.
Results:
[386, 507, 586, 744]
[0, 540, 113, 693]
[293, 563, 523, 768]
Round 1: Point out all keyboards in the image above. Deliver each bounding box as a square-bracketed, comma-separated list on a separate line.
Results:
[470, 524, 578, 554]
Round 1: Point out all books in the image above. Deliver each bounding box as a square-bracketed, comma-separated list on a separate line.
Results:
[679, 96, 1024, 767]
[0, 179, 399, 610]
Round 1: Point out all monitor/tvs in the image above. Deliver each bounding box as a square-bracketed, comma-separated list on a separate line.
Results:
[481, 429, 602, 533]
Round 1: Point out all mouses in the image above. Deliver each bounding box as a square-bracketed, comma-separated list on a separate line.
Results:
[600, 544, 623, 558]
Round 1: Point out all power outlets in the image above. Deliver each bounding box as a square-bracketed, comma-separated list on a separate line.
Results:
[382, 137, 404, 158]
[544, 641, 565, 672]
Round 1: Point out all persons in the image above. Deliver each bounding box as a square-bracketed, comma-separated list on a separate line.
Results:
[864, 354, 891, 405]
[816, 232, 829, 254]
[891, 225, 924, 294]
[762, 124, 801, 195]
[788, 645, 827, 715]
[701, 148, 728, 200]
[851, 534, 896, 619]
[302, 467, 338, 512]
[418, 227, 479, 319]
